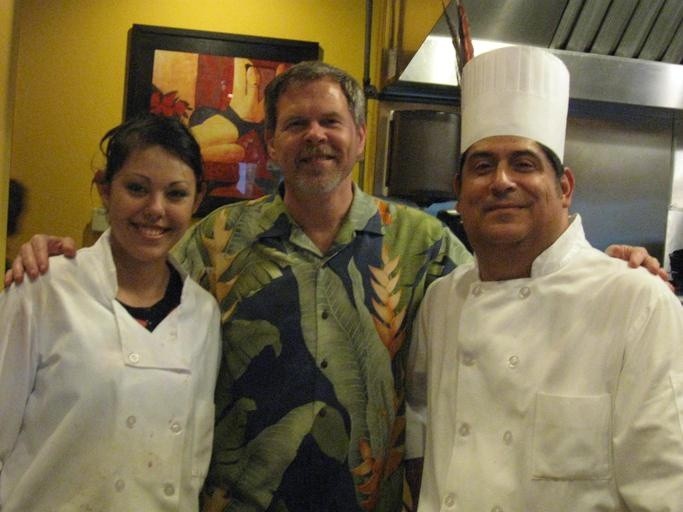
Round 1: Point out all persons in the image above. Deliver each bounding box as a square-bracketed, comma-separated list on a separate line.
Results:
[4, 59, 675, 512]
[405, 136, 683, 512]
[190, 58, 285, 164]
[0, 115, 223, 512]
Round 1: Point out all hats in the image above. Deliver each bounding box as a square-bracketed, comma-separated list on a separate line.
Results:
[460, 45, 570, 164]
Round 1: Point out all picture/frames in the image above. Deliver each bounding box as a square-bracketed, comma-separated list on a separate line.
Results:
[123, 24, 322, 223]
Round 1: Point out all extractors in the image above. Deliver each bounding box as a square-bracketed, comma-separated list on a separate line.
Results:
[390, 0, 683, 112]
